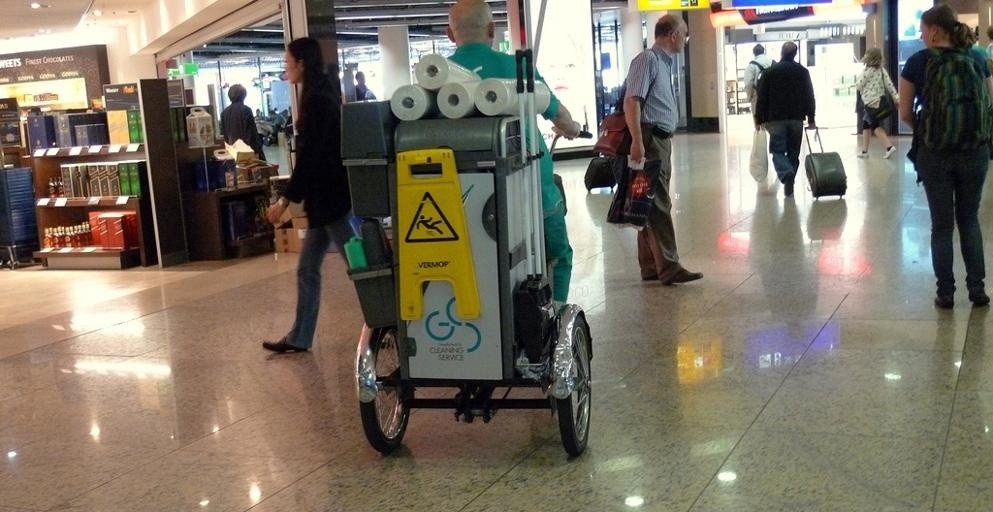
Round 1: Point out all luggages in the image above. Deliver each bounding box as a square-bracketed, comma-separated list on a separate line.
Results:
[804, 127, 847, 203]
[584, 157, 618, 195]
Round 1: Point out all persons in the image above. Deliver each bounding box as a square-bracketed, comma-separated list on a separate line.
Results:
[899, 5, 993, 309]
[745, 44, 779, 134]
[856, 49, 900, 159]
[972, 45, 990, 69]
[985, 24, 993, 59]
[622, 14, 702, 286]
[447, 0, 580, 304]
[755, 42, 817, 194]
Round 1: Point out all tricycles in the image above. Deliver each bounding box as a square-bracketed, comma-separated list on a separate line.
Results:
[342, 49, 596, 458]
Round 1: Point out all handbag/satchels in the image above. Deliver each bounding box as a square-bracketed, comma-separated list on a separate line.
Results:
[865, 90, 896, 126]
[593, 108, 629, 155]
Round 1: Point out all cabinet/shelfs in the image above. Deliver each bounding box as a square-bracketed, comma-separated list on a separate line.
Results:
[724, 67, 755, 114]
[168, 79, 279, 261]
[1, 97, 39, 269]
[26, 78, 189, 270]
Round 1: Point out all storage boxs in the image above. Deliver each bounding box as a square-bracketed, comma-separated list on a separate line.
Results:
[345, 263, 397, 328]
[264, 182, 342, 256]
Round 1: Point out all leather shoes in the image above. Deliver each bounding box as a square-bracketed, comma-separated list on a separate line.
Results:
[260, 333, 309, 355]
[661, 268, 704, 284]
[641, 273, 661, 281]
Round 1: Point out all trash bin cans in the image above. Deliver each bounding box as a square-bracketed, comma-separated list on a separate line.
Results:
[387, 117, 543, 380]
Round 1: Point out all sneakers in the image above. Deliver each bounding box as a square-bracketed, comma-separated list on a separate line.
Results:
[967, 288, 990, 307]
[784, 173, 796, 197]
[883, 146, 896, 160]
[857, 151, 869, 159]
[933, 288, 955, 310]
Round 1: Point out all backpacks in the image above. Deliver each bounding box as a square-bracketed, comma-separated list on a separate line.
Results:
[922, 46, 992, 156]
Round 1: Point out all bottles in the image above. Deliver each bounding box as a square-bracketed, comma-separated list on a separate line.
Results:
[360, 217, 388, 267]
[47, 176, 65, 198]
[342, 237, 369, 271]
[41, 219, 92, 250]
